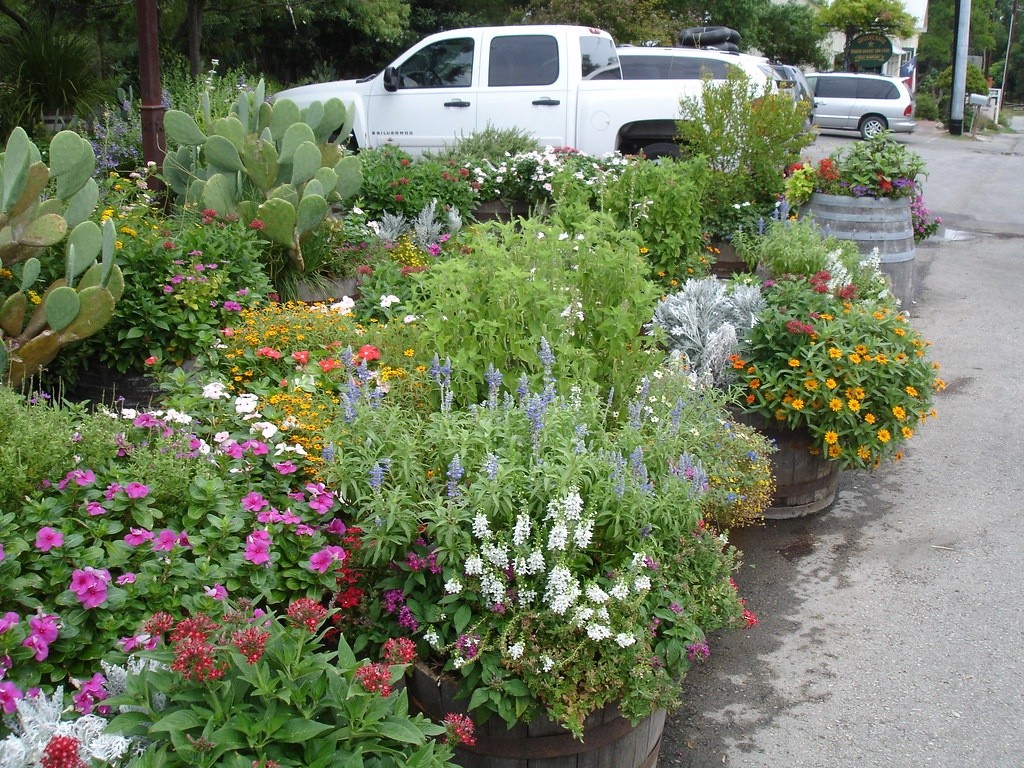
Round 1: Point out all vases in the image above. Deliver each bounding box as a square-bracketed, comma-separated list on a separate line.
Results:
[285, 275, 360, 309]
[404, 658, 668, 768]
[795, 193, 915, 311]
[704, 244, 751, 282]
[463, 200, 528, 230]
[722, 393, 842, 520]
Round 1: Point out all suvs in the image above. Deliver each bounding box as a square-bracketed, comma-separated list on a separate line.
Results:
[806, 72, 917, 141]
[542, 44, 817, 130]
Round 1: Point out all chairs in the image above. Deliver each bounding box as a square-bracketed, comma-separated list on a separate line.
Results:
[508, 50, 534, 84]
[489, 49, 512, 86]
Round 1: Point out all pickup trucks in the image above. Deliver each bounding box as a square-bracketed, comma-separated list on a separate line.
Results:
[266, 25, 778, 169]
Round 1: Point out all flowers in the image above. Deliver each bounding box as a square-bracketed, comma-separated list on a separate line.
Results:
[0, 56, 949, 768]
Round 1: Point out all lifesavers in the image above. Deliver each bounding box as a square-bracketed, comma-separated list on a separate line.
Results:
[675, 25, 741, 55]
